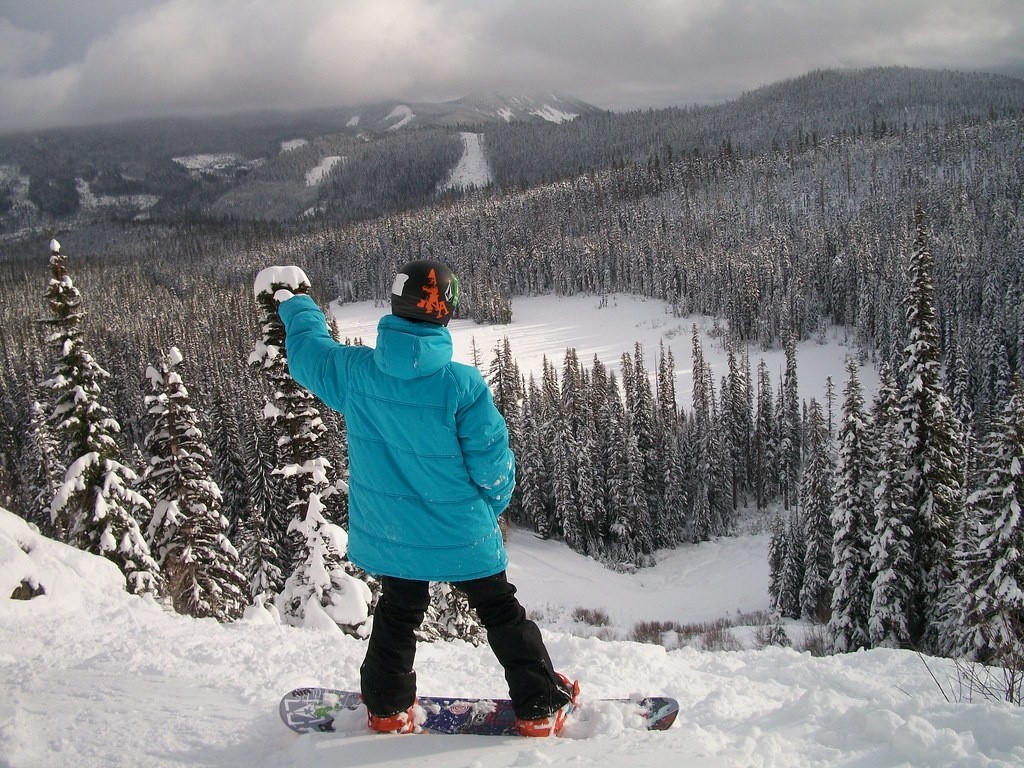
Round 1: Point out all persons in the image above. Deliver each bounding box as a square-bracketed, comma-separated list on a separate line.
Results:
[277, 259, 580, 737]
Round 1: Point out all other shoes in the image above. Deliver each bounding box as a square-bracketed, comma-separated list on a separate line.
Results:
[367, 694, 419, 733]
[514, 672, 580, 737]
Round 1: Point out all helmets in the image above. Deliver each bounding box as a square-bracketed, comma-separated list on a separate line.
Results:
[391, 260, 459, 327]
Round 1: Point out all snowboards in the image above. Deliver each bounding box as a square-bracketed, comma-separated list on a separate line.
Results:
[278, 687, 680, 737]
